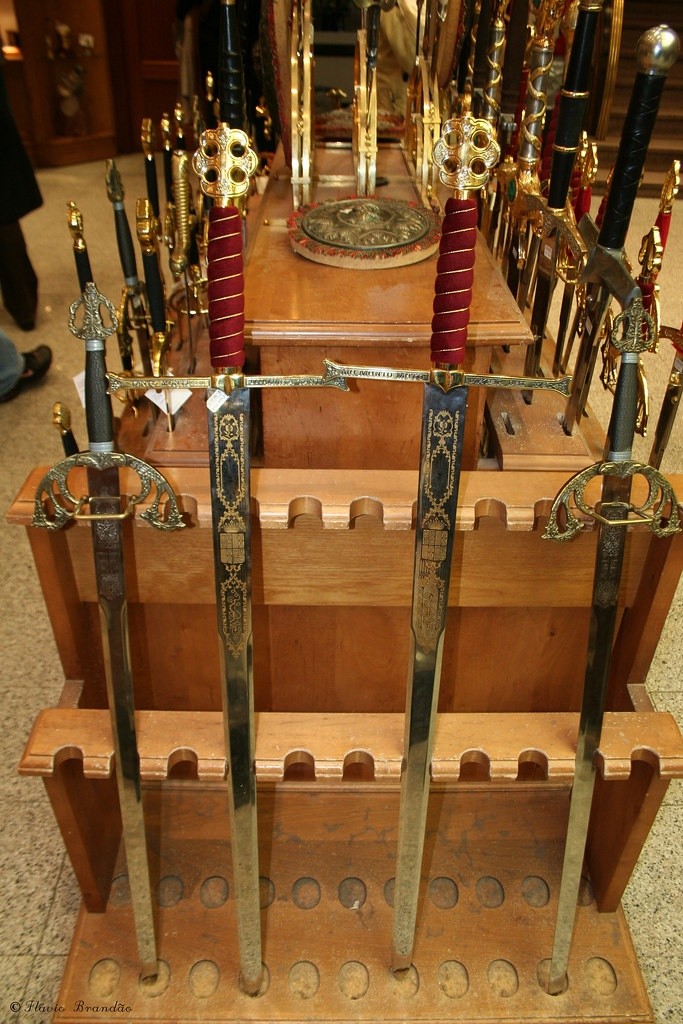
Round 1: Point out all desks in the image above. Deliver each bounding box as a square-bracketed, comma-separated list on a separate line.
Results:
[245, 137, 535, 470]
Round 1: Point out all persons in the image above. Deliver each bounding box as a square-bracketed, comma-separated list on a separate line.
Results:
[174, 0, 270, 151]
[0, 51, 44, 331]
[0, 332, 52, 403]
[375, 0, 427, 116]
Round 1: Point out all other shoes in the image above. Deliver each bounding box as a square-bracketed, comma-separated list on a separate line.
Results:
[14, 276, 38, 331]
[0, 344, 51, 404]
